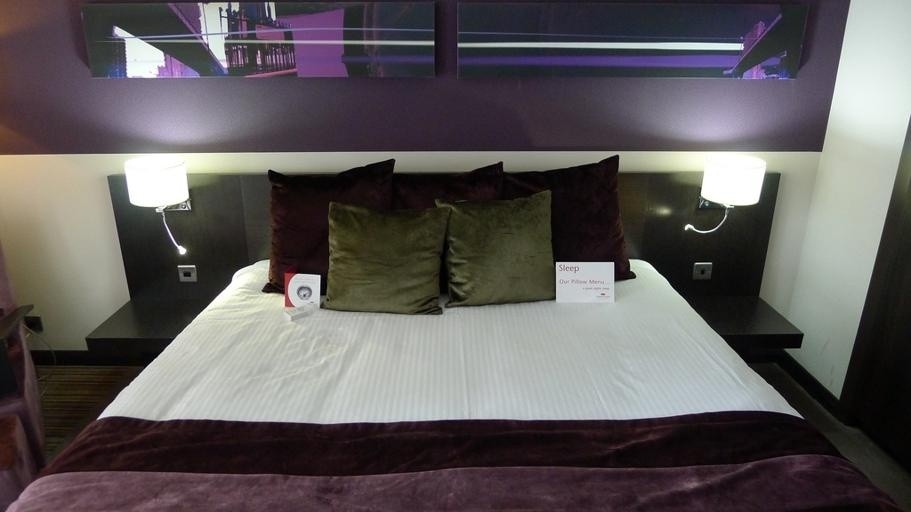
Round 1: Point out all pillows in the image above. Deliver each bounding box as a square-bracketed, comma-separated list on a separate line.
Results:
[435, 189, 556, 308]
[319, 201, 451, 315]
[262, 159, 396, 296]
[503, 154, 636, 282]
[393, 161, 503, 210]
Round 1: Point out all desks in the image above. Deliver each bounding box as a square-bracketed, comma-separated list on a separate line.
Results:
[684, 297, 804, 373]
[85, 299, 214, 366]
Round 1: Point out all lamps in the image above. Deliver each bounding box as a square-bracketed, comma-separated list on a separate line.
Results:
[124, 153, 192, 255]
[685, 151, 767, 234]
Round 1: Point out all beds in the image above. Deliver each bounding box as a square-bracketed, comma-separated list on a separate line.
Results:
[4, 170, 907, 512]
